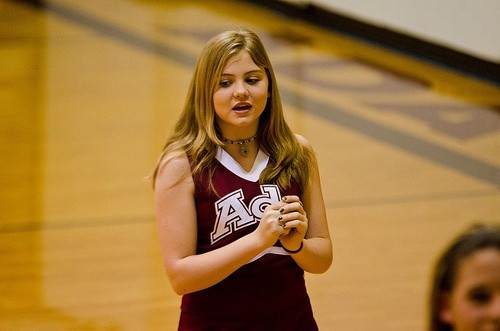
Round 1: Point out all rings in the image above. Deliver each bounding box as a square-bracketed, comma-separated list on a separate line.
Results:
[299, 201, 304, 206]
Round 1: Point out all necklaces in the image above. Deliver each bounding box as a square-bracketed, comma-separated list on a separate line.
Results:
[217, 135, 260, 157]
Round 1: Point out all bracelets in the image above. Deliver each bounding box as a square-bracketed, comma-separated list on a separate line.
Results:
[277, 239, 304, 254]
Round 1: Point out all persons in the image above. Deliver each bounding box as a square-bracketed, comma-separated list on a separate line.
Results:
[430, 230, 500, 331]
[153, 29, 334, 331]
